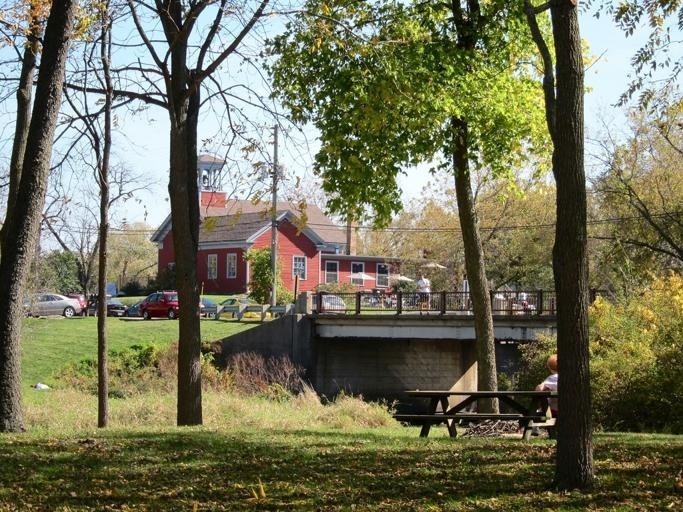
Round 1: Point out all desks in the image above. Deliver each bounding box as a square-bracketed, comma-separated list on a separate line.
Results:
[401, 388, 558, 439]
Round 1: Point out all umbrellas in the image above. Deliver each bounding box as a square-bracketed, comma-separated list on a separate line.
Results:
[421, 261, 447, 272]
[346, 272, 377, 290]
[386, 275, 414, 283]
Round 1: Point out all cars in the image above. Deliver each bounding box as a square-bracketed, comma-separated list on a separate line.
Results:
[137, 290, 205, 320]
[21, 293, 82, 318]
[198, 297, 216, 317]
[287, 294, 347, 316]
[67, 294, 87, 316]
[125, 302, 141, 318]
[208, 299, 262, 321]
[83, 294, 127, 318]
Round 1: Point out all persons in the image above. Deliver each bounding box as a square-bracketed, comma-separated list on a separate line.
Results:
[418, 274, 431, 315]
[519, 290, 527, 310]
[527, 353, 559, 419]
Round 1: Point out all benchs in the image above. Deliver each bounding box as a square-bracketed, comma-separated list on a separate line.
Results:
[394, 415, 559, 442]
